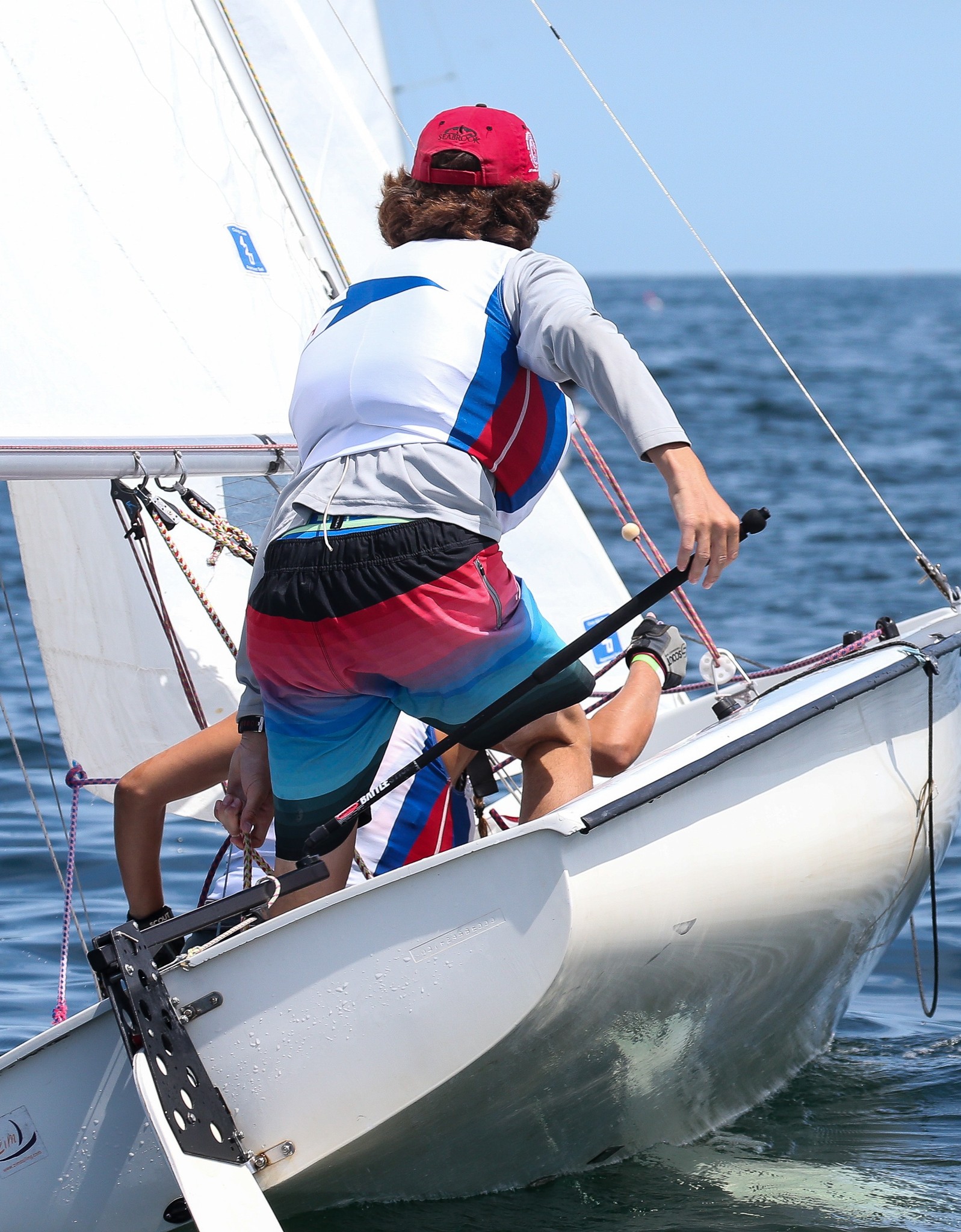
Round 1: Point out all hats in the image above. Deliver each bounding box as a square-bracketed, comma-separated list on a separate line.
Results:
[410, 103, 539, 186]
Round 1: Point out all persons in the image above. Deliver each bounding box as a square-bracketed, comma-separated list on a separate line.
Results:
[114, 611, 689, 965]
[214, 104, 740, 926]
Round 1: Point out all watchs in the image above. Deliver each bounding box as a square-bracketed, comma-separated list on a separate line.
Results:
[238, 715, 264, 733]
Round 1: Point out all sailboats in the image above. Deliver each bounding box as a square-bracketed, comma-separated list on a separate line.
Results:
[0, 0, 961, 1232]
